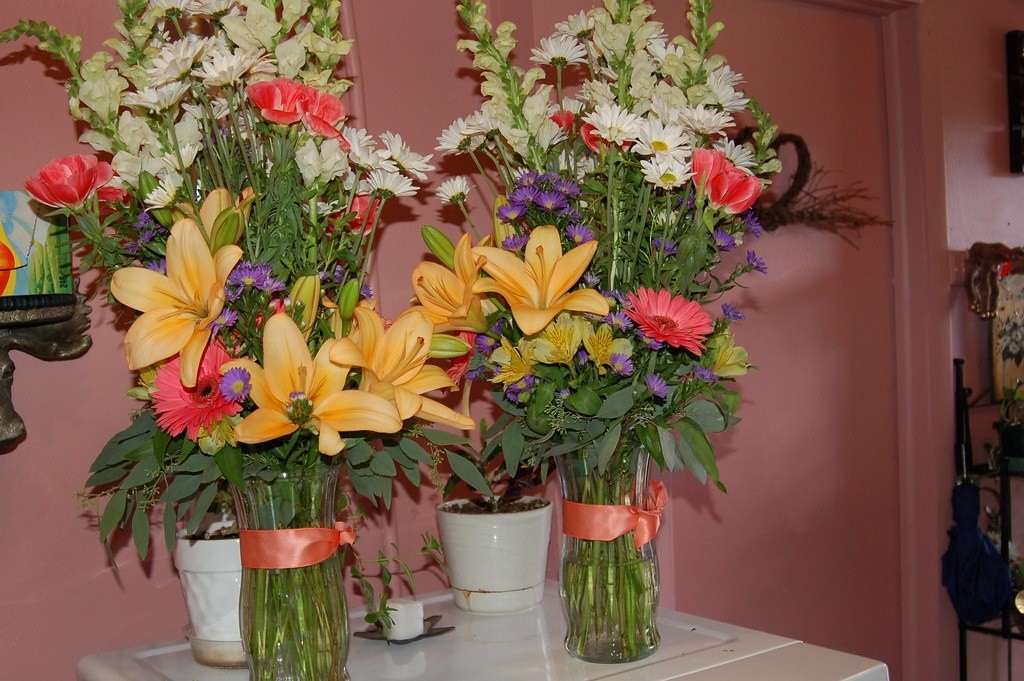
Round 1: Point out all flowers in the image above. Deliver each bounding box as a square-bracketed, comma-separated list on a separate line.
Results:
[0, 0, 782, 642]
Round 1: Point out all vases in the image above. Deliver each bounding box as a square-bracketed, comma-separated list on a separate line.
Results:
[164, 522, 242, 668]
[232, 474, 349, 680]
[549, 445, 660, 662]
[434, 498, 553, 612]
